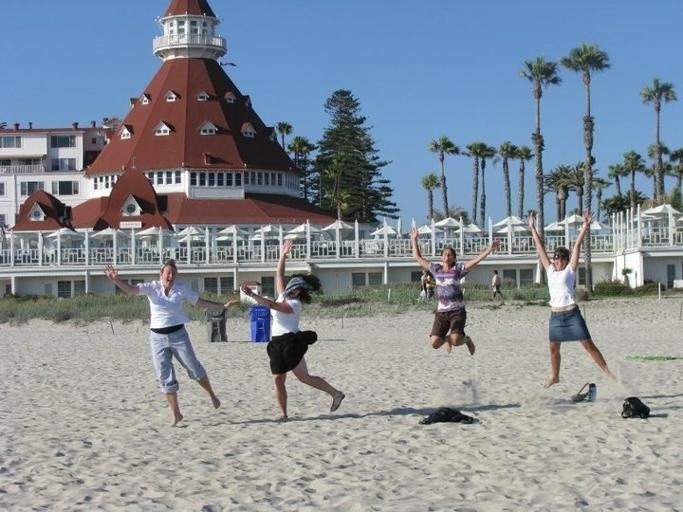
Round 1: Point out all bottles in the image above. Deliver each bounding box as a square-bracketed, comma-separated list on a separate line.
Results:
[588, 383, 596, 401]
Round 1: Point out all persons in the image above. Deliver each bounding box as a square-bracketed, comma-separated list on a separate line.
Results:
[421, 270, 428, 299]
[527, 207, 618, 389]
[241, 239, 345, 422]
[489, 270, 503, 301]
[104, 258, 241, 427]
[426, 270, 436, 301]
[409, 227, 501, 356]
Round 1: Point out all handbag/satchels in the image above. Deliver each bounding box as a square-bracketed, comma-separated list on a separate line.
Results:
[621, 397, 650, 418]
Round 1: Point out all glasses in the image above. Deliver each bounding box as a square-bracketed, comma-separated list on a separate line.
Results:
[554, 254, 568, 259]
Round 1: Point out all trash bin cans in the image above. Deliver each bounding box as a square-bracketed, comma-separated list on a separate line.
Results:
[204, 308, 228, 343]
[248, 304, 271, 342]
